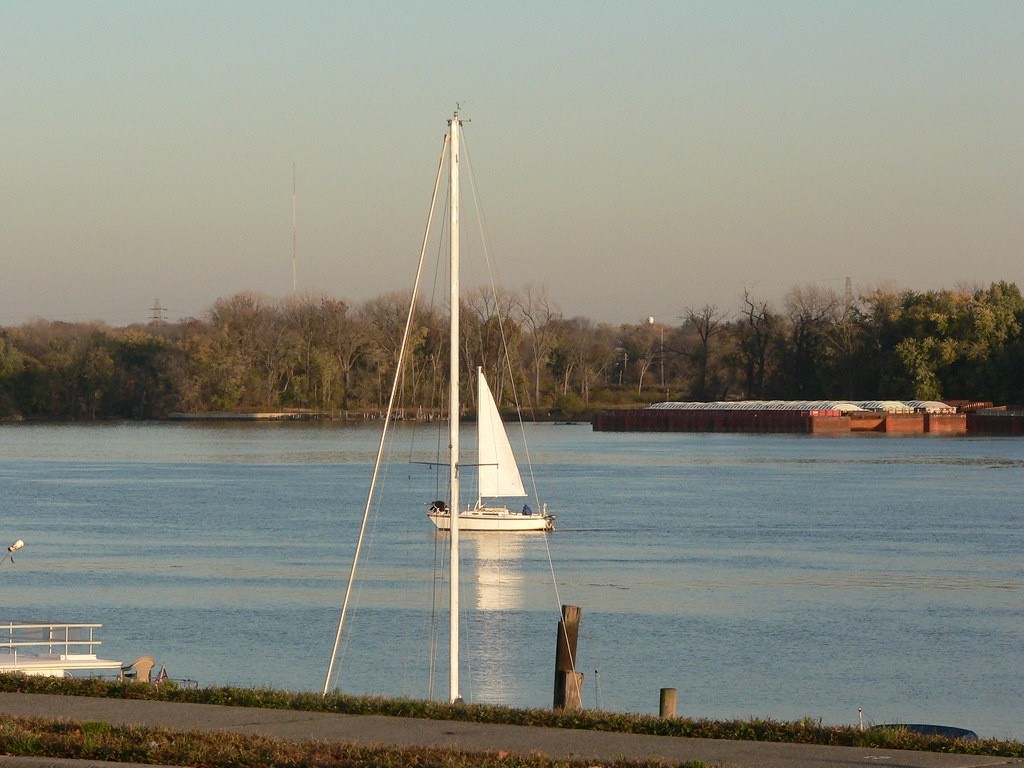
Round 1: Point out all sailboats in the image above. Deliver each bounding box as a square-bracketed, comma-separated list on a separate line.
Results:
[424, 366, 556, 531]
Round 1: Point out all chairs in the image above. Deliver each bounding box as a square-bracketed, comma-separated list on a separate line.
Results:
[120, 655, 155, 684]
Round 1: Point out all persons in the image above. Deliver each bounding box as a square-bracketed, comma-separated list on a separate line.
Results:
[430, 501, 448, 514]
[522, 504, 532, 515]
